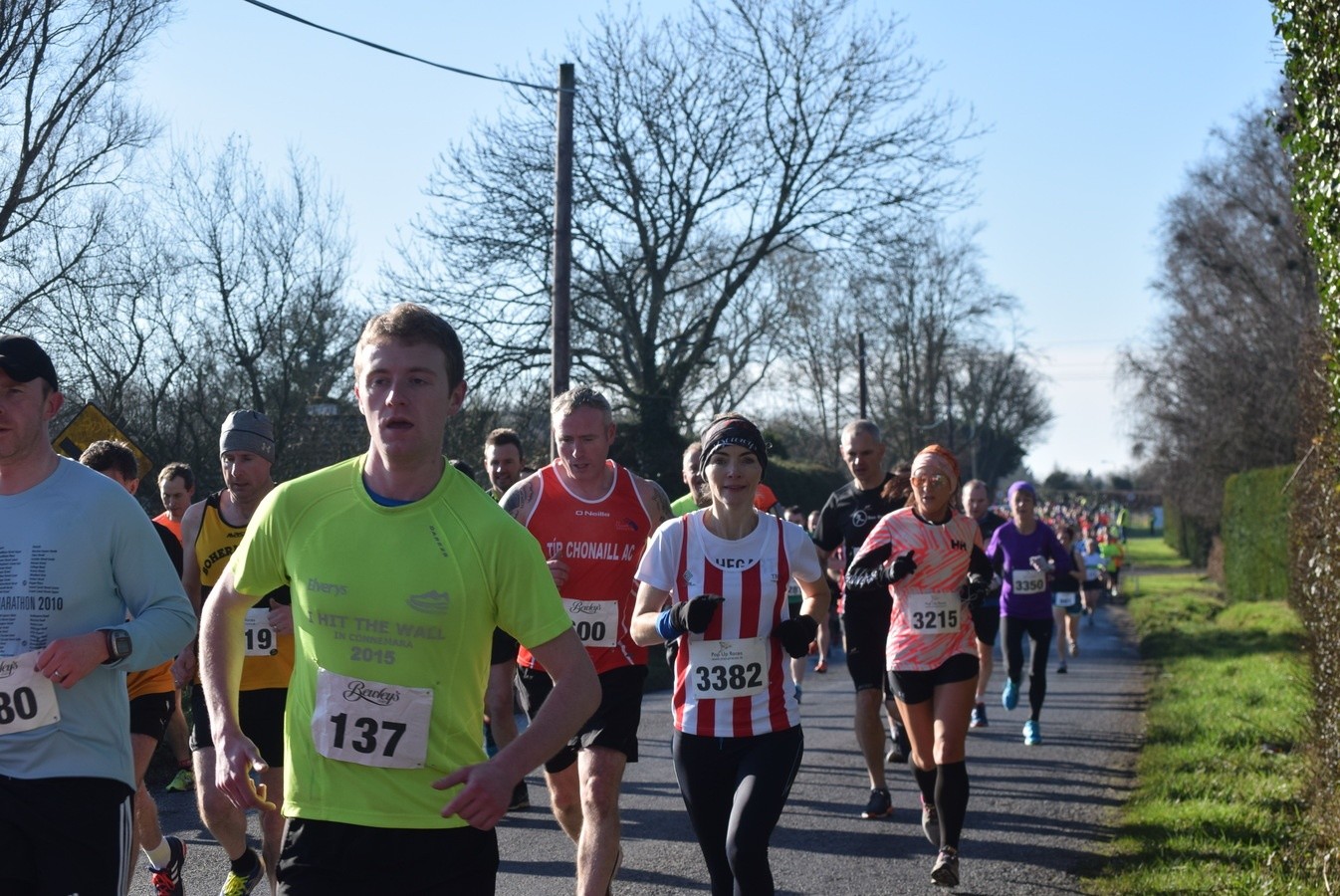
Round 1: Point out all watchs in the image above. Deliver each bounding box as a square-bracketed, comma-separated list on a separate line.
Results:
[95, 627, 133, 665]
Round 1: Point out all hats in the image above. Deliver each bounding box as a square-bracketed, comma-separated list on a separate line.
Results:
[0, 334, 57, 391]
[220, 410, 275, 464]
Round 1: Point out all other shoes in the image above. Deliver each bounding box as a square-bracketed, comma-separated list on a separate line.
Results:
[1069, 640, 1079, 657]
[1057, 660, 1068, 673]
[507, 781, 530, 809]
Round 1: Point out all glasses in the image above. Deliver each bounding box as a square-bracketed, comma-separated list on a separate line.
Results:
[910, 475, 949, 488]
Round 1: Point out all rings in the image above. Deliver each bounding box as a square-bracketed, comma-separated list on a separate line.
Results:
[54, 670, 63, 680]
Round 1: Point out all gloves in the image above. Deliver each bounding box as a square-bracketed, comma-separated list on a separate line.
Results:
[962, 583, 985, 616]
[881, 550, 917, 582]
[671, 594, 725, 634]
[775, 615, 818, 660]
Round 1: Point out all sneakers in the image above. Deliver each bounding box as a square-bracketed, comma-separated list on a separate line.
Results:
[149, 835, 186, 896]
[859, 786, 892, 819]
[219, 855, 264, 896]
[920, 791, 941, 846]
[1022, 720, 1040, 746]
[166, 768, 197, 792]
[930, 850, 960, 888]
[970, 703, 988, 728]
[1003, 669, 1024, 709]
[886, 738, 911, 763]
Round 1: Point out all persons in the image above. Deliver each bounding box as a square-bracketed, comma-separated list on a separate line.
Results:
[198, 303, 602, 896]
[80, 407, 296, 896]
[0, 331, 197, 896]
[449, 386, 1129, 896]
